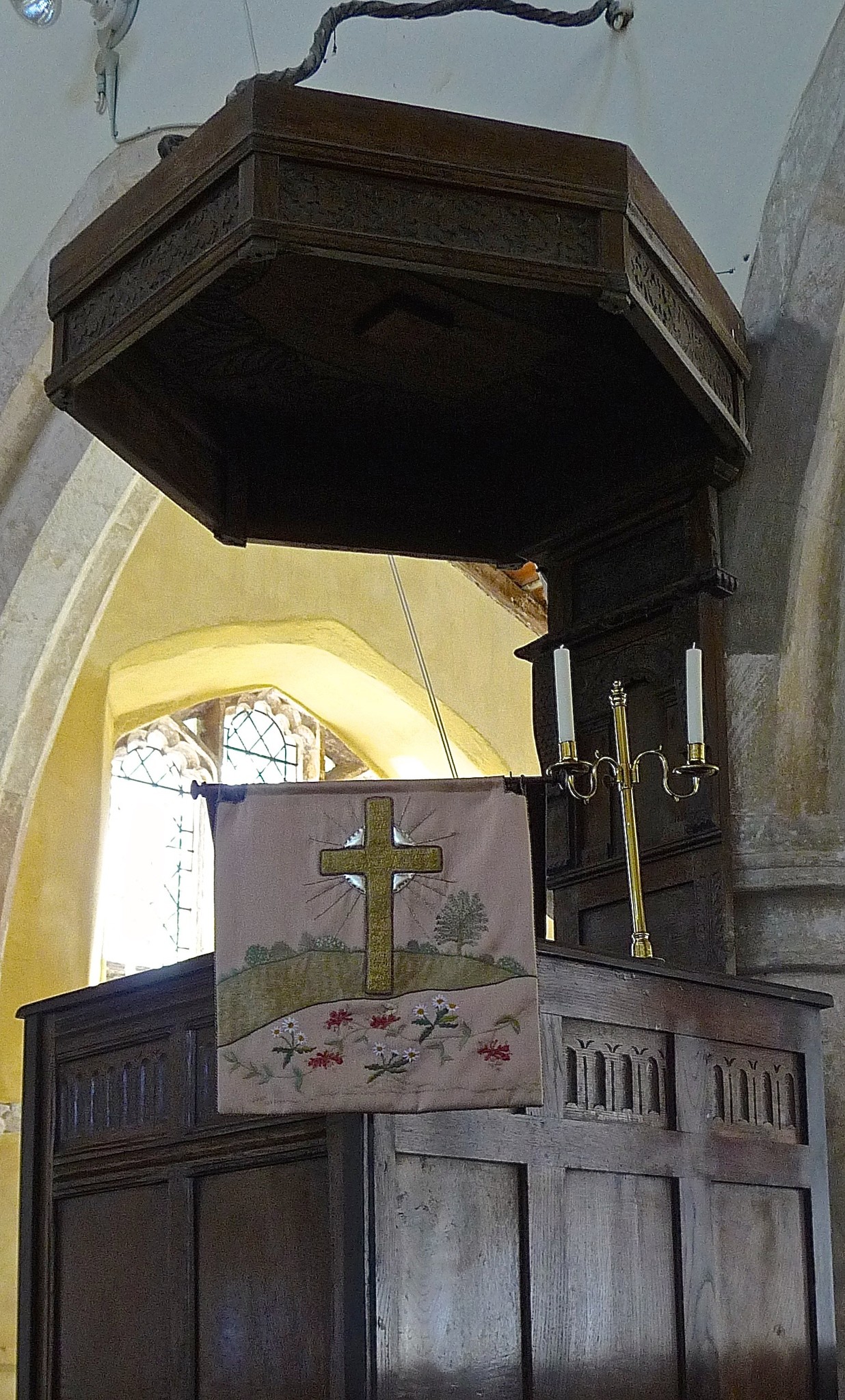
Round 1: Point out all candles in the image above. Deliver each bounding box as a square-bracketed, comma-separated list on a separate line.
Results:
[551, 637, 577, 747]
[678, 636, 713, 748]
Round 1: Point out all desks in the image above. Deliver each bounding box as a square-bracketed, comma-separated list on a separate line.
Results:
[8, 922, 845, 1400]
